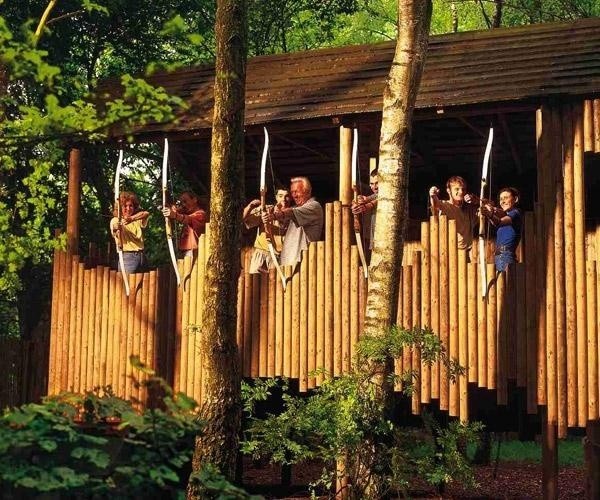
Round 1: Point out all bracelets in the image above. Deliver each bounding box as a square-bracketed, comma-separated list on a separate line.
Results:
[489, 213, 494, 220]
[176, 213, 178, 218]
[281, 211, 285, 219]
[273, 214, 277, 219]
[370, 202, 376, 208]
[363, 204, 367, 210]
[181, 214, 185, 223]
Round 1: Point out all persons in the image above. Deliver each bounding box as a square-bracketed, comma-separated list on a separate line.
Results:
[241, 182, 292, 274]
[428, 175, 480, 264]
[481, 187, 524, 275]
[349, 169, 379, 254]
[260, 175, 324, 268]
[107, 191, 150, 273]
[161, 191, 208, 262]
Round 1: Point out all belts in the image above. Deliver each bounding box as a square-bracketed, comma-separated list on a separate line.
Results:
[122, 250, 146, 253]
[499, 244, 508, 250]
